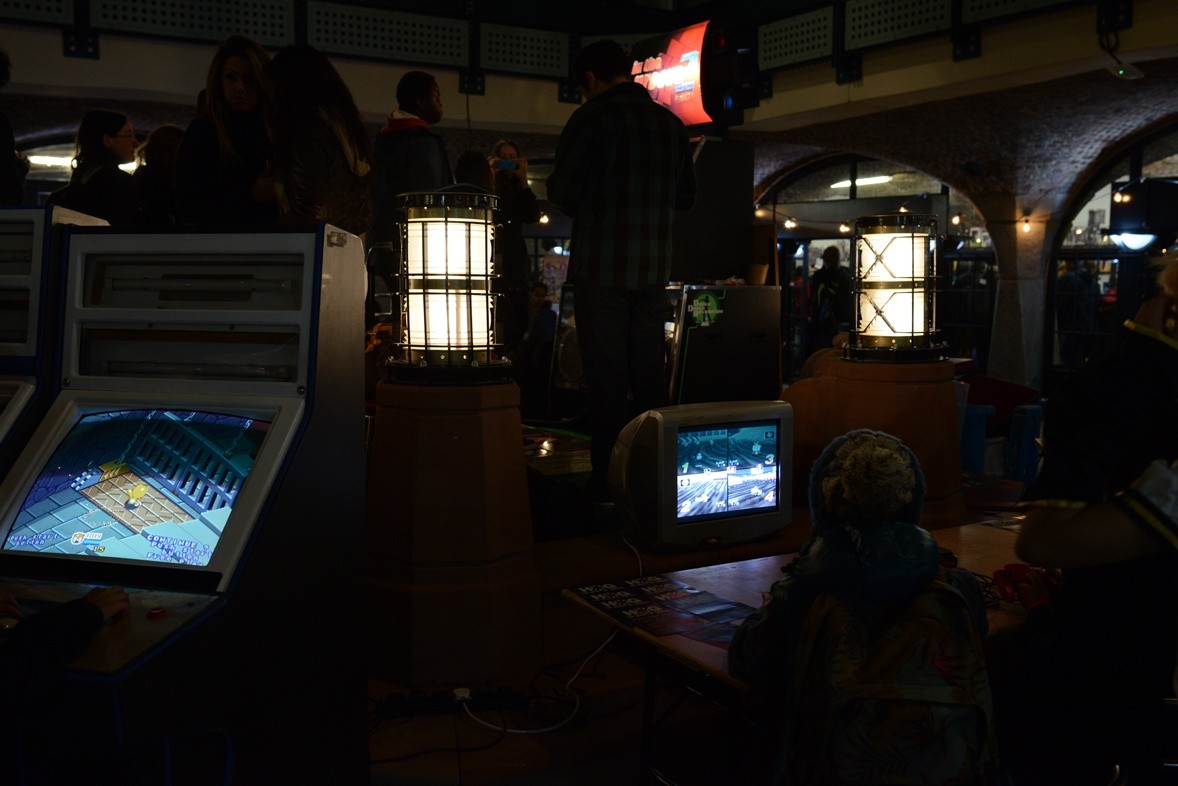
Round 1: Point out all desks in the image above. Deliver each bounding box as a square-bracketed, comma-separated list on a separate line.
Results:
[563, 511, 1066, 786]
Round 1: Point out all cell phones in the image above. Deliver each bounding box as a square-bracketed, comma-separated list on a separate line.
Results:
[496, 160, 518, 171]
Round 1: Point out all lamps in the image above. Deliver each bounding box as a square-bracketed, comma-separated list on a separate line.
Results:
[388, 186, 507, 380]
[837, 204, 950, 365]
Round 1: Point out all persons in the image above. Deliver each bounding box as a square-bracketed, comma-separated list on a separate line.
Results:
[728, 429, 1000, 786]
[1014, 248, 1178, 786]
[0, 578, 131, 786]
[4, 28, 1175, 490]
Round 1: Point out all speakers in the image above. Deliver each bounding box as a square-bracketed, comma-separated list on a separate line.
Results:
[1112, 179, 1177, 234]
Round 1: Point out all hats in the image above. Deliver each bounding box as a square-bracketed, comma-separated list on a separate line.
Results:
[807, 428, 929, 528]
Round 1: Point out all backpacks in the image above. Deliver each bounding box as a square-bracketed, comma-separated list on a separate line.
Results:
[781, 553, 1001, 786]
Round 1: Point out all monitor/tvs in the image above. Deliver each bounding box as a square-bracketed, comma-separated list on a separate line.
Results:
[657, 402, 793, 547]
[622, 18, 715, 133]
[669, 283, 785, 401]
[0, 387, 310, 606]
